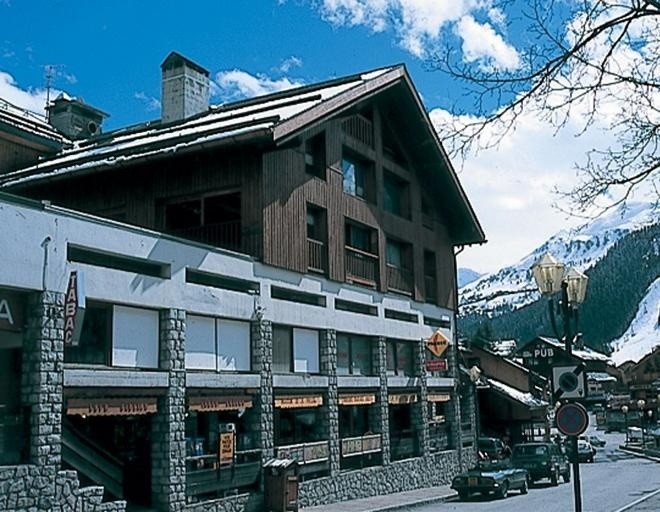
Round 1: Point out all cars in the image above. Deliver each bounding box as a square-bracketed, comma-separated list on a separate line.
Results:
[564, 435, 607, 464]
[452, 458, 530, 499]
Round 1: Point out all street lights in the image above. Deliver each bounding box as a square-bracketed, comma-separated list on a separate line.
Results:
[638, 400, 646, 448]
[530, 250, 590, 511]
[622, 404, 629, 444]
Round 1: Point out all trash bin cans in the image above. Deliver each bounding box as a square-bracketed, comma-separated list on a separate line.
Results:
[263, 458, 299, 511]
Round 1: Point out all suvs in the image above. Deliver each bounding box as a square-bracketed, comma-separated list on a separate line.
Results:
[479, 438, 511, 460]
[512, 442, 571, 486]
